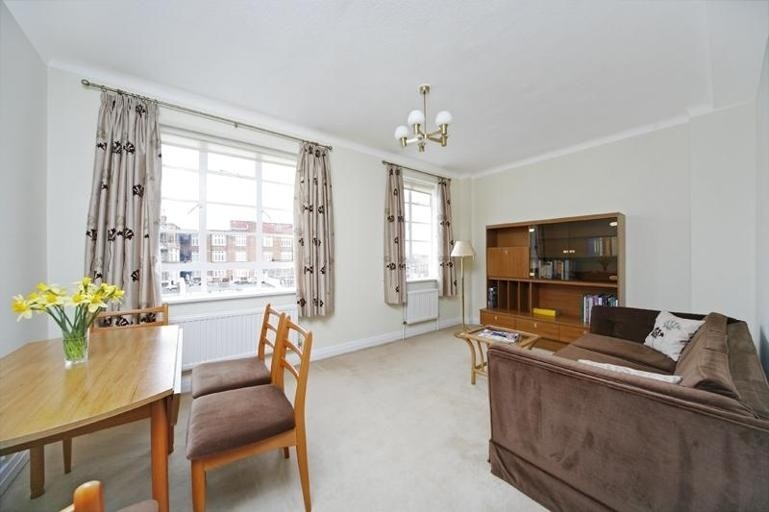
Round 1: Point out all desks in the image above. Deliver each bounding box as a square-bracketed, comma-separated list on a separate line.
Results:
[0, 324, 181, 512]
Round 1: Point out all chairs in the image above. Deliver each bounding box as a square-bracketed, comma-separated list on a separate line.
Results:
[185, 316, 313, 512]
[58, 480, 160, 512]
[89, 304, 169, 330]
[191, 303, 286, 399]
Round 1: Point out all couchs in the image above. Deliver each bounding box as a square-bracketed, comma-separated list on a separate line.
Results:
[486, 305, 769, 512]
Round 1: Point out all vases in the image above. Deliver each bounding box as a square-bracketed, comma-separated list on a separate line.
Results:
[63, 337, 88, 369]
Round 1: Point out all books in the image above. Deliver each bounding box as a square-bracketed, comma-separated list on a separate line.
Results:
[530, 257, 575, 281]
[583, 294, 618, 330]
[487, 285, 497, 311]
[479, 328, 520, 343]
[533, 307, 561, 317]
[586, 236, 619, 256]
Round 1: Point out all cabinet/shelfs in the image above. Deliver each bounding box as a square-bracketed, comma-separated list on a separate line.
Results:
[479, 212, 625, 328]
[480, 307, 591, 344]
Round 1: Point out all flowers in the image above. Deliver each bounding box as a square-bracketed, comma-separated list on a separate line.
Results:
[10, 275, 126, 362]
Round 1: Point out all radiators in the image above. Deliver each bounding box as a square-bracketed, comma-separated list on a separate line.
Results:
[403, 288, 439, 325]
[169, 303, 298, 374]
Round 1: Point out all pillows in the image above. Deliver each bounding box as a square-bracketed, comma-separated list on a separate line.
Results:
[577, 359, 682, 385]
[672, 312, 742, 401]
[643, 309, 706, 363]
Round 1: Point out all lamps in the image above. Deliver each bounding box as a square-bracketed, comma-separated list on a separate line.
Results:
[451, 240, 476, 338]
[393, 84, 453, 154]
